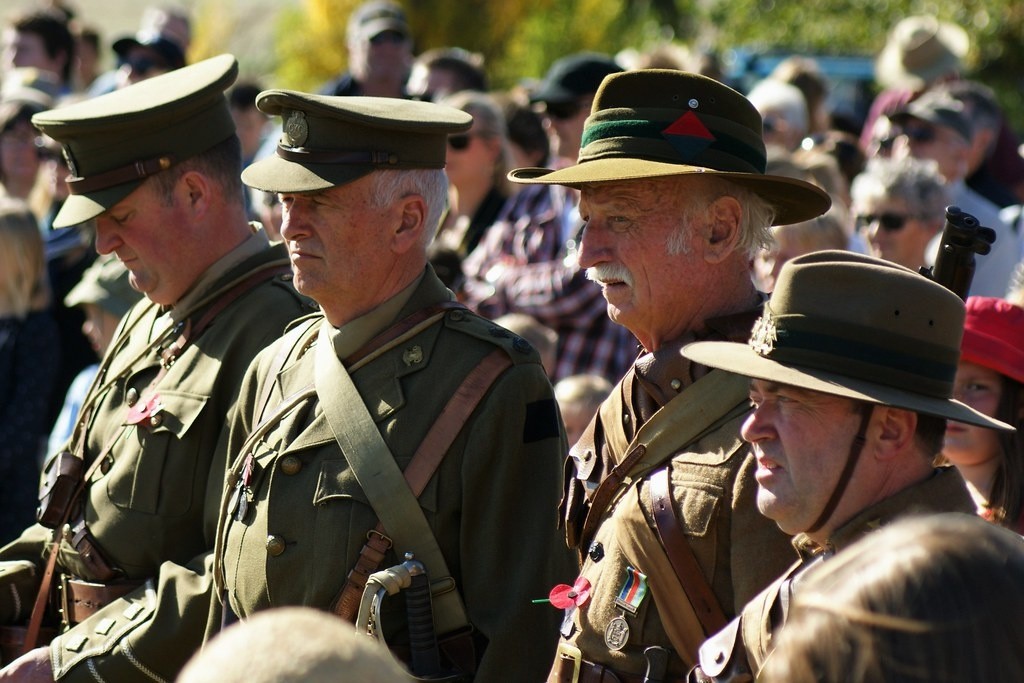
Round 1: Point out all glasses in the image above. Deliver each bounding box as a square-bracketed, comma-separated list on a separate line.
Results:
[855, 211, 916, 231]
[446, 130, 492, 149]
[763, 115, 781, 134]
[547, 102, 591, 119]
[117, 56, 158, 75]
[880, 126, 934, 148]
[371, 34, 406, 45]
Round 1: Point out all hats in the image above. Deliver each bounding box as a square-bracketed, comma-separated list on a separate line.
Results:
[112, 31, 186, 71]
[875, 13, 969, 91]
[240, 89, 474, 193]
[31, 53, 239, 230]
[961, 296, 1024, 382]
[507, 69, 832, 226]
[679, 249, 1016, 432]
[65, 251, 145, 317]
[528, 52, 625, 105]
[352, 4, 409, 38]
[888, 106, 974, 142]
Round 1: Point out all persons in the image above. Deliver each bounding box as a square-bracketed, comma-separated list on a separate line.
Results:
[0, 1, 1024, 683]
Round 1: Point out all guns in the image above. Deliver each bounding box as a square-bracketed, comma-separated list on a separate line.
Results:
[919, 205, 999, 298]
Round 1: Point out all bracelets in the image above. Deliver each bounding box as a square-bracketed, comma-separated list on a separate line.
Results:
[485, 262, 507, 283]
